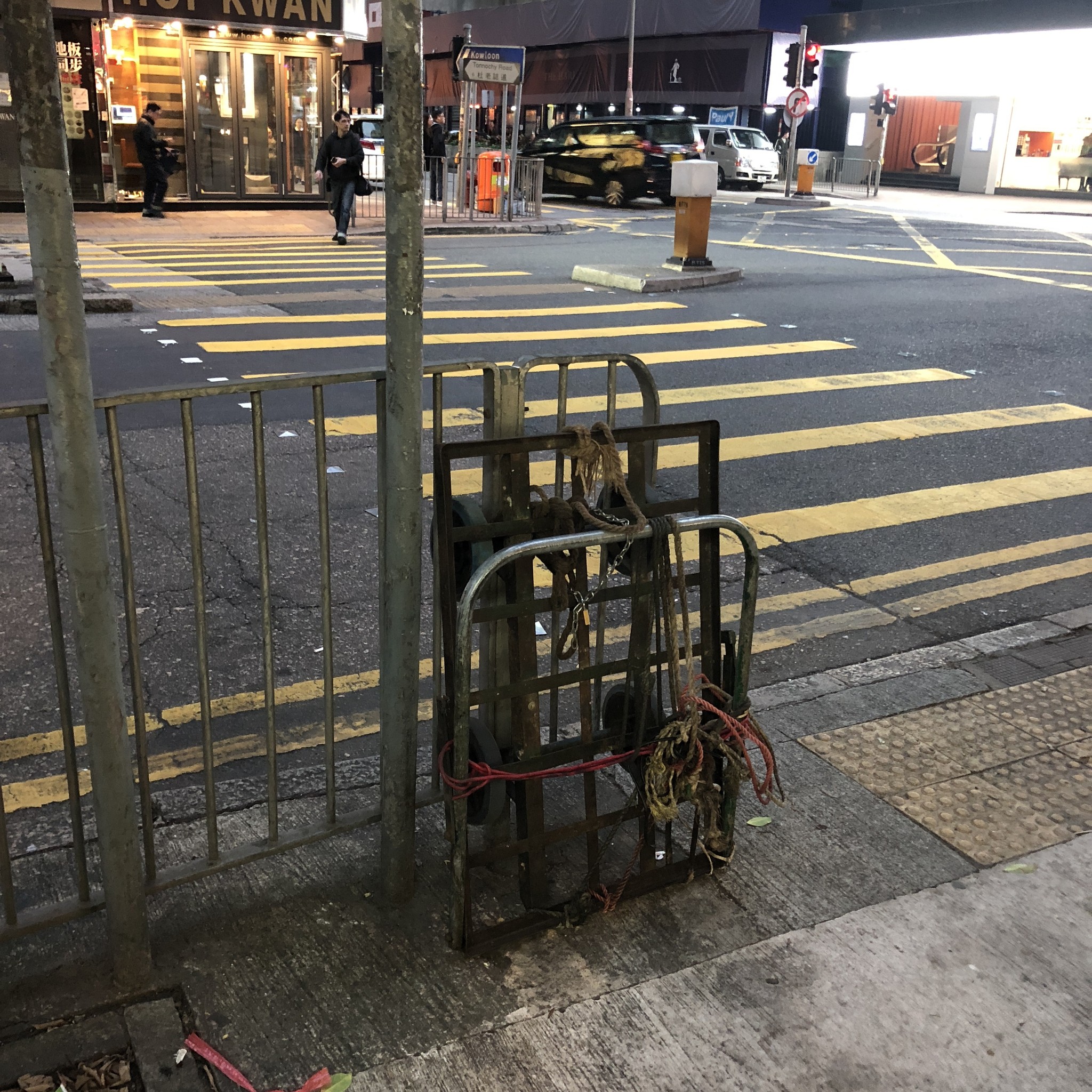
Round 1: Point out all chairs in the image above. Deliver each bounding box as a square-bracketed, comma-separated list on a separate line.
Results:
[119, 138, 146, 192]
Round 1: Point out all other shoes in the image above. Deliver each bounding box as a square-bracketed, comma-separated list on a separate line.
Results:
[142, 213, 154, 217]
[437, 201, 442, 206]
[148, 207, 164, 219]
[295, 178, 300, 184]
[332, 234, 338, 241]
[302, 181, 305, 185]
[338, 237, 347, 245]
[430, 199, 437, 205]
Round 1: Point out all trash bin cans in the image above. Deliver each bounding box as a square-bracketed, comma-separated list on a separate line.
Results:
[476, 151, 513, 213]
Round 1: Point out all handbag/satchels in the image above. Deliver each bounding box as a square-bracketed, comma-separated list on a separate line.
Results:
[354, 175, 374, 196]
[160, 147, 183, 176]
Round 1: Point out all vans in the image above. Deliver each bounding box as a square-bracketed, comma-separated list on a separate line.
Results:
[348, 115, 779, 207]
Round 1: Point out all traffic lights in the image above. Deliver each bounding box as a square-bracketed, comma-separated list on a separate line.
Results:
[783, 43, 800, 87]
[883, 86, 901, 115]
[870, 80, 884, 116]
[803, 43, 821, 88]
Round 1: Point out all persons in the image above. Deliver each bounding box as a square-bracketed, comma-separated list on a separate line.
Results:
[132, 103, 174, 219]
[428, 110, 447, 207]
[422, 111, 434, 206]
[293, 117, 312, 184]
[775, 131, 795, 181]
[314, 110, 364, 245]
